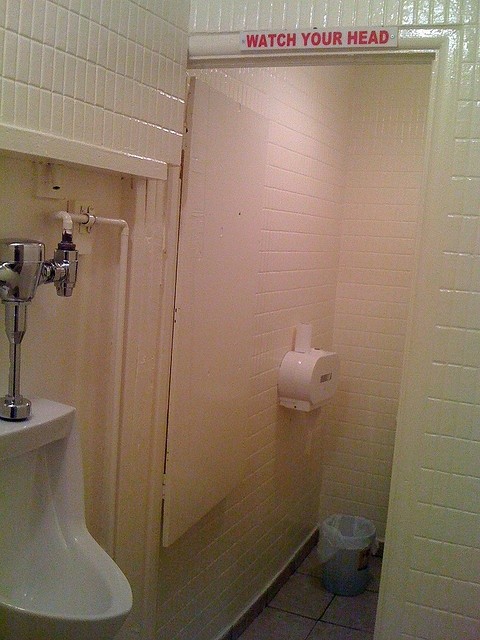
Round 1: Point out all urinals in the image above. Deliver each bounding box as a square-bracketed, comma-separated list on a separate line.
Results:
[0, 398, 137, 639]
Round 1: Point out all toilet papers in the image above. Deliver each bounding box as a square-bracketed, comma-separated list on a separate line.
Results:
[294, 321, 311, 350]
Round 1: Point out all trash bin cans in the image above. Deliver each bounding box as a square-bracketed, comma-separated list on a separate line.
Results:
[317, 514, 379, 596]
